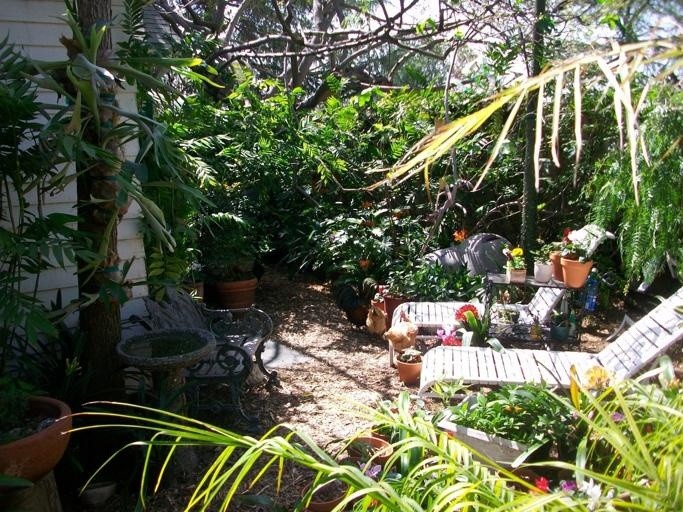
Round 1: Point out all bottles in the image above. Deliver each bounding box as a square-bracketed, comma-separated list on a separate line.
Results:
[583, 267, 598, 315]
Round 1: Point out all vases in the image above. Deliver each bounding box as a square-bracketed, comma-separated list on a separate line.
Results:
[506, 268, 526, 284]
[549, 250, 563, 281]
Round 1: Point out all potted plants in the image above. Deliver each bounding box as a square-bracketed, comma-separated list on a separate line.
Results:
[530, 245, 553, 283]
[394, 346, 423, 385]
[551, 310, 578, 341]
[560, 243, 592, 288]
[206, 202, 419, 328]
[302, 376, 579, 512]
[0, 0, 221, 487]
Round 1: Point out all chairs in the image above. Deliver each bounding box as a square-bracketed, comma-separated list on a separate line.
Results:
[144, 286, 283, 436]
[418, 286, 683, 404]
[389, 225, 615, 366]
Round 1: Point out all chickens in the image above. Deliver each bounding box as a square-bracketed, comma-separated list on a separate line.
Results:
[364, 300, 387, 335]
[382, 309, 419, 355]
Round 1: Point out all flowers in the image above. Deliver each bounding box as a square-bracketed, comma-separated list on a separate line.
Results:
[552, 228, 572, 250]
[502, 244, 527, 270]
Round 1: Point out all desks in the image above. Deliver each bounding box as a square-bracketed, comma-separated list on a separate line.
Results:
[487, 273, 592, 352]
